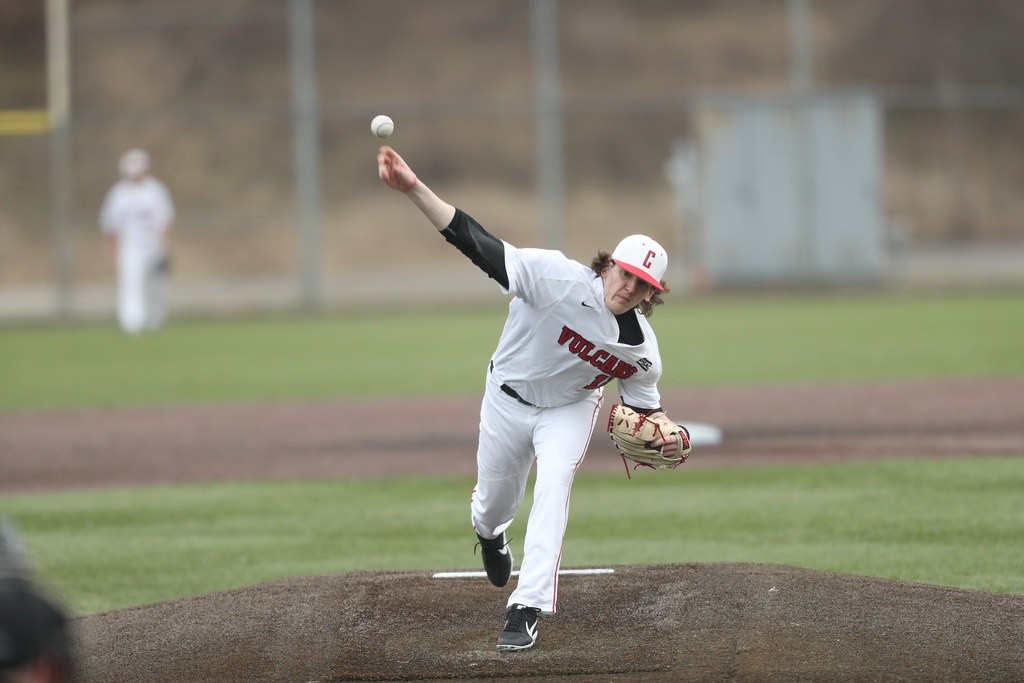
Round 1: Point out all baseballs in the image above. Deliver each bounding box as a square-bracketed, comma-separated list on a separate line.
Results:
[370, 115, 395, 138]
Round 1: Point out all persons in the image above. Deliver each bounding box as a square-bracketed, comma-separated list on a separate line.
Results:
[97, 148, 177, 334]
[376, 144, 693, 650]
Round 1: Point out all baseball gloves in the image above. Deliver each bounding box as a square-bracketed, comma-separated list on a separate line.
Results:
[606, 403, 694, 472]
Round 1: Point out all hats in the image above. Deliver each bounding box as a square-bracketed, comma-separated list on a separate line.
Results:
[612, 234, 668, 291]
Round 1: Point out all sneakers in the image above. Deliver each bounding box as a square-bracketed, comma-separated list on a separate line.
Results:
[474, 531, 513, 588]
[497, 603, 541, 650]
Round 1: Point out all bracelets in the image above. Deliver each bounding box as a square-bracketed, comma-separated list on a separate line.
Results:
[400, 173, 417, 193]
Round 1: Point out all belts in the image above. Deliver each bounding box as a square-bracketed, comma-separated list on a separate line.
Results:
[489, 361, 533, 406]
